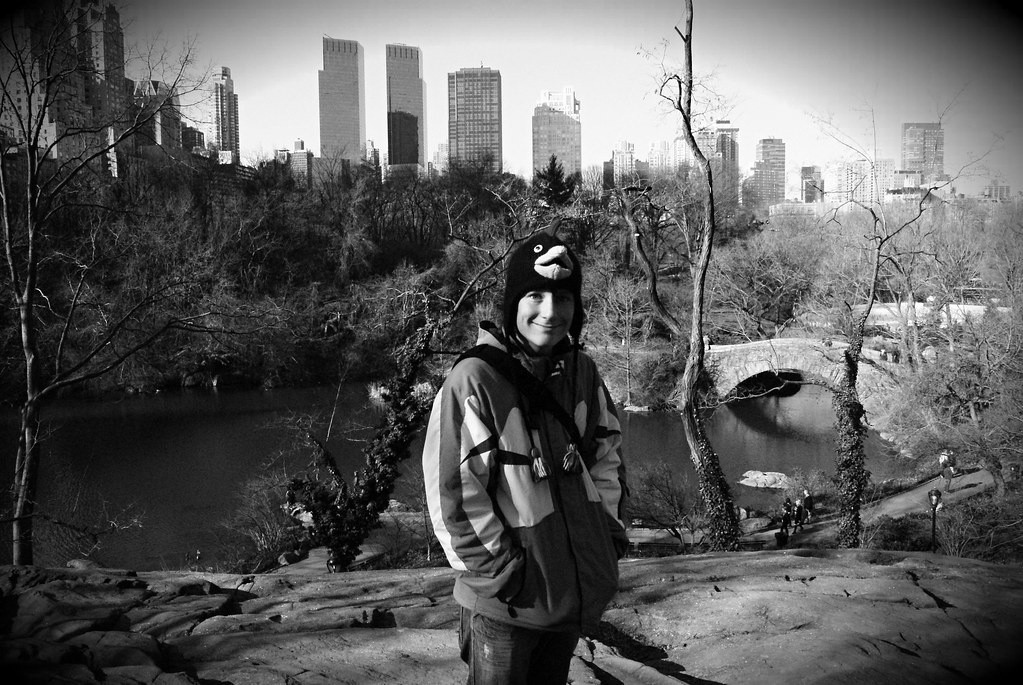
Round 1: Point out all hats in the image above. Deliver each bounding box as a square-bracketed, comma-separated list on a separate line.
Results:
[503, 219, 583, 482]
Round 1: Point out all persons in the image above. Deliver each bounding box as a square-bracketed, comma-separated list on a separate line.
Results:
[781, 508, 789, 535]
[792, 501, 803, 534]
[804, 489, 819, 524]
[941, 450, 957, 494]
[422, 231, 632, 685]
[784, 498, 792, 529]
